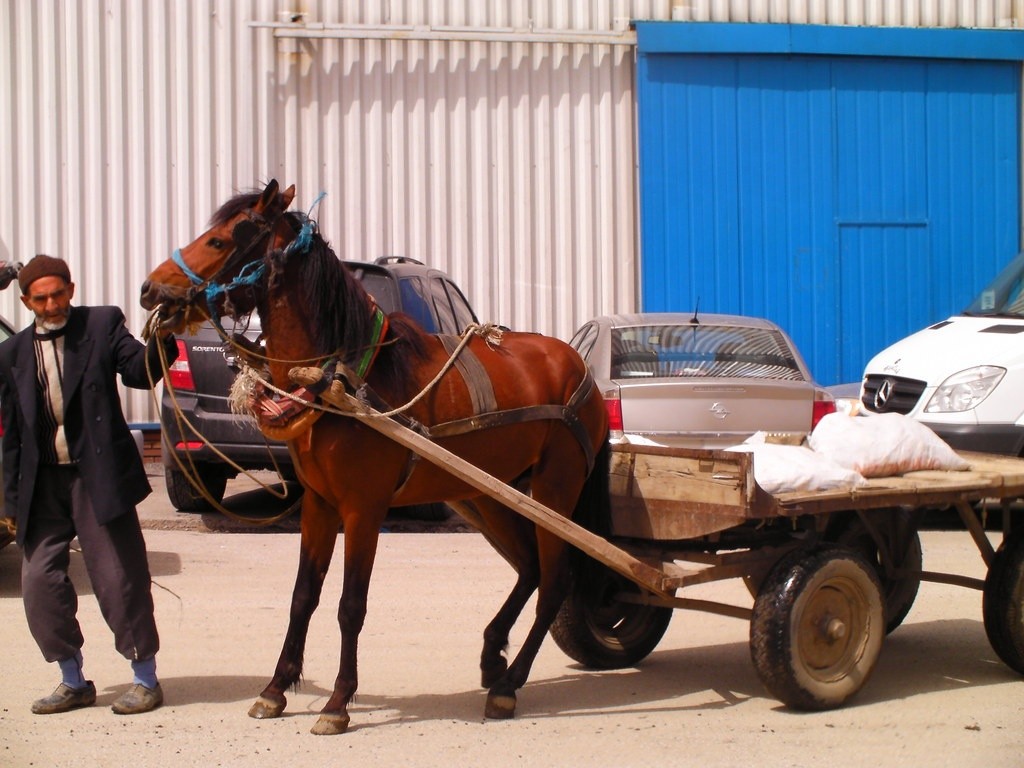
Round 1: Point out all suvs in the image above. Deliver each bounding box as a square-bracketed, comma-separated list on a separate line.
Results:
[161, 252, 503, 530]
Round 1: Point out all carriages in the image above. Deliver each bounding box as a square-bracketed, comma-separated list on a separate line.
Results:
[136, 178, 1024, 735]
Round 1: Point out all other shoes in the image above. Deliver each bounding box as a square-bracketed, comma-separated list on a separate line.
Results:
[29, 680, 165, 713]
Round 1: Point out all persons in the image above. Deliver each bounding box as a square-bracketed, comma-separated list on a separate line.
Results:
[1, 256, 181, 713]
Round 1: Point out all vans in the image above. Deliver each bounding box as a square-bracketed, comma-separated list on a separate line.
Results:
[855, 250, 1023, 435]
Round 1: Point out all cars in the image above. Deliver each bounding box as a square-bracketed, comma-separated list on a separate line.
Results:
[565, 295, 839, 446]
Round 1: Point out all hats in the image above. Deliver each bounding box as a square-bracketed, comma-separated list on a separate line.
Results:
[18, 254, 71, 295]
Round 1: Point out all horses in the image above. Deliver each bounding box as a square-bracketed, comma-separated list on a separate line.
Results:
[139, 178, 614, 737]
